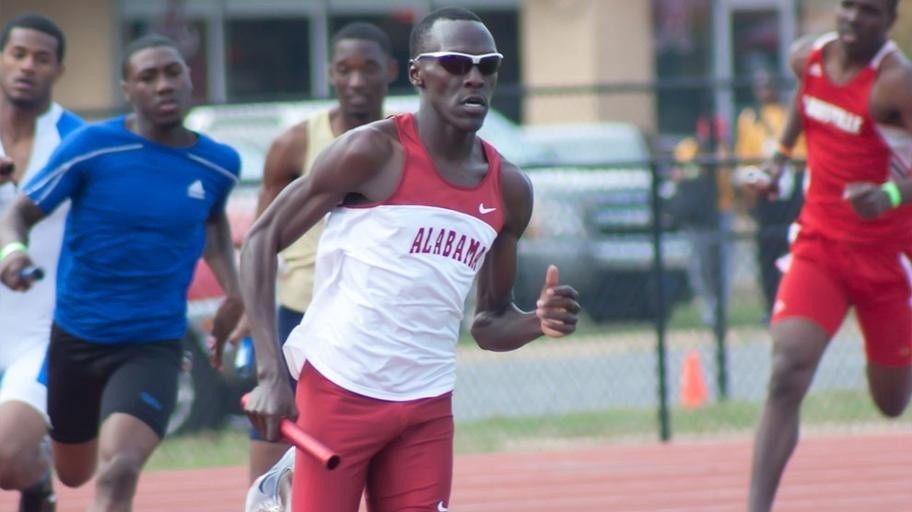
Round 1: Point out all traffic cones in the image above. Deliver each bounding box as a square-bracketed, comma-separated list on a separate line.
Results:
[679, 344, 708, 406]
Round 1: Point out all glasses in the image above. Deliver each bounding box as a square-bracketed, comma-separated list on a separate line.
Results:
[414, 51, 505, 77]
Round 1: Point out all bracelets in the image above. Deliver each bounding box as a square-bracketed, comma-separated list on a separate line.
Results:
[0, 241, 27, 261]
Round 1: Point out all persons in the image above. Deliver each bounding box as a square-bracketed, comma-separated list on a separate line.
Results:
[0, 16, 89, 512]
[247, 19, 401, 489]
[0, 33, 245, 512]
[239, 7, 581, 512]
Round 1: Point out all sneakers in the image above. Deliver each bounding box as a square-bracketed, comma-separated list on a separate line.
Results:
[244, 445, 297, 510]
[18, 441, 55, 512]
